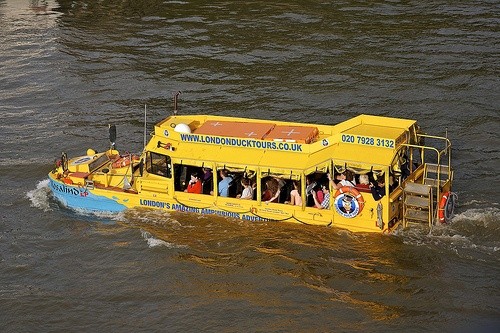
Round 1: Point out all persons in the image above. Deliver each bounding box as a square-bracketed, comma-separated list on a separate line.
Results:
[210, 169, 257, 201]
[156, 159, 171, 178]
[184, 172, 203, 194]
[306, 173, 331, 209]
[262, 179, 302, 206]
[326, 169, 356, 190]
[202, 167, 211, 180]
[357, 155, 415, 200]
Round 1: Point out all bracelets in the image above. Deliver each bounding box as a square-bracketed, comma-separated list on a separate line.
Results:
[329, 179, 331, 181]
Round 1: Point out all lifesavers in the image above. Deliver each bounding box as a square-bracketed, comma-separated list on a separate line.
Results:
[334, 187, 364, 218]
[439, 191, 455, 225]
[112, 156, 139, 168]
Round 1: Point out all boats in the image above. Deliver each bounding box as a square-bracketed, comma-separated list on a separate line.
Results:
[49, 91, 459, 236]
